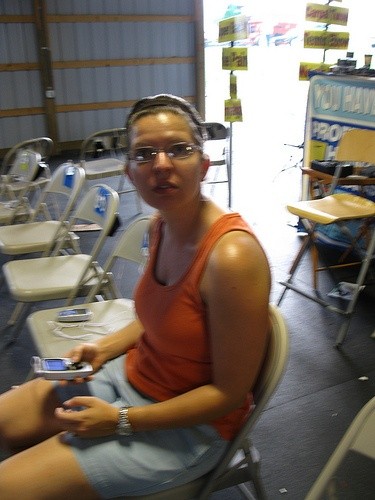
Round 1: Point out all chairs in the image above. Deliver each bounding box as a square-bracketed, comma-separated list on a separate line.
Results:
[305, 398, 375, 500]
[201, 121, 231, 209]
[278, 129, 375, 347]
[0, 129, 152, 383]
[135, 304, 287, 500]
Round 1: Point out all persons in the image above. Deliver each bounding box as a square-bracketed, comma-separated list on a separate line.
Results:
[0, 93, 272, 500]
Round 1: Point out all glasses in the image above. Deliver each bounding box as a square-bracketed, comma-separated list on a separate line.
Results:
[127, 141, 202, 164]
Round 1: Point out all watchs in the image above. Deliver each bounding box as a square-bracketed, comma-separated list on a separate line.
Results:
[133, 323, 185, 436]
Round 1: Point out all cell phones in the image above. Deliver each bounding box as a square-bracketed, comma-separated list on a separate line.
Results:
[31, 356, 93, 375]
[55, 306, 92, 322]
